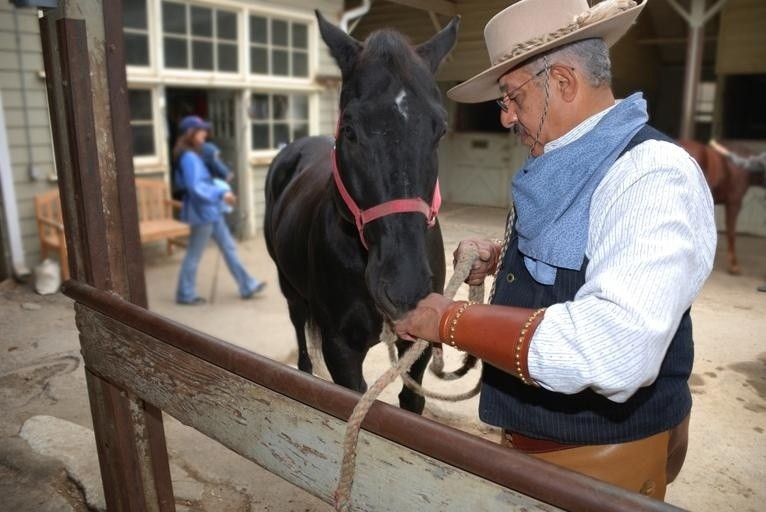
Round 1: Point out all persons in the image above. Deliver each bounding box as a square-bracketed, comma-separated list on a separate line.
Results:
[394, 1, 718, 506]
[174, 123, 235, 187]
[172, 116, 268, 306]
[708, 136, 765, 293]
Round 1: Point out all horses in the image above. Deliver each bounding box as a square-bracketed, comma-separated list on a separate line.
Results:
[263, 8, 462, 416]
[675, 138, 766, 275]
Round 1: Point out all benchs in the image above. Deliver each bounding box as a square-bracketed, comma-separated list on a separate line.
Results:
[34, 177, 191, 281]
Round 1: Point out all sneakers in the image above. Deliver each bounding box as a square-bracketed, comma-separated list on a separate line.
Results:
[177, 281, 268, 304]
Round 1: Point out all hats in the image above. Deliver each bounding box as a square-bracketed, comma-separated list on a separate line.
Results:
[445, 0, 648, 107]
[179, 116, 213, 132]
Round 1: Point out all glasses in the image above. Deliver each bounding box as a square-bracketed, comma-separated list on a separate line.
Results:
[496, 64, 576, 114]
